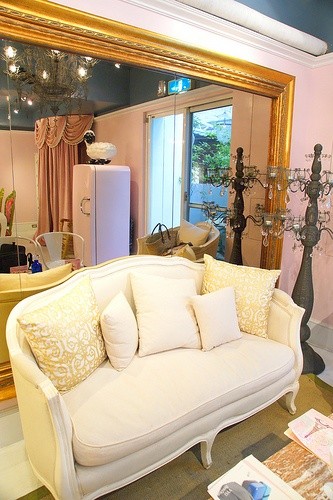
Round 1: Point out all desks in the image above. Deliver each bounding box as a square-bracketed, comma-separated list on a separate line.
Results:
[208, 413, 333, 500]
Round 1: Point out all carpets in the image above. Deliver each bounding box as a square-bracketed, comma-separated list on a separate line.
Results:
[15, 372, 333, 500]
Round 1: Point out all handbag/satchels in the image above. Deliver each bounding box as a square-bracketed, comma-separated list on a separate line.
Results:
[146, 223, 178, 255]
[161, 241, 196, 261]
[0, 242, 27, 273]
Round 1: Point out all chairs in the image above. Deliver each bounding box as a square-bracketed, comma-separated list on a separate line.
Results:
[59, 218, 74, 259]
[35, 231, 85, 272]
[0, 235, 45, 274]
[137, 217, 220, 262]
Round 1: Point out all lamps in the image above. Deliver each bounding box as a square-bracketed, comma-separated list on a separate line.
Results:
[0, 38, 121, 137]
[254, 143, 333, 377]
[200, 147, 267, 266]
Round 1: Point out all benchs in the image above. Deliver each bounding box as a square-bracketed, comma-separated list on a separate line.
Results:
[0, 254, 205, 364]
[5, 254, 307, 500]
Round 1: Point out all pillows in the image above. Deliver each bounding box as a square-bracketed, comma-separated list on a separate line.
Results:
[16, 275, 108, 396]
[174, 243, 197, 261]
[129, 271, 202, 358]
[99, 290, 139, 373]
[201, 254, 282, 339]
[175, 217, 211, 247]
[27, 263, 72, 288]
[0, 273, 29, 292]
[189, 284, 244, 352]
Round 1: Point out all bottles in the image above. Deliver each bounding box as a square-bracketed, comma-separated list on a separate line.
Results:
[31, 255, 42, 273]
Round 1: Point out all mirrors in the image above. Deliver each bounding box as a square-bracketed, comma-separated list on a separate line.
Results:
[0, 0, 298, 400]
